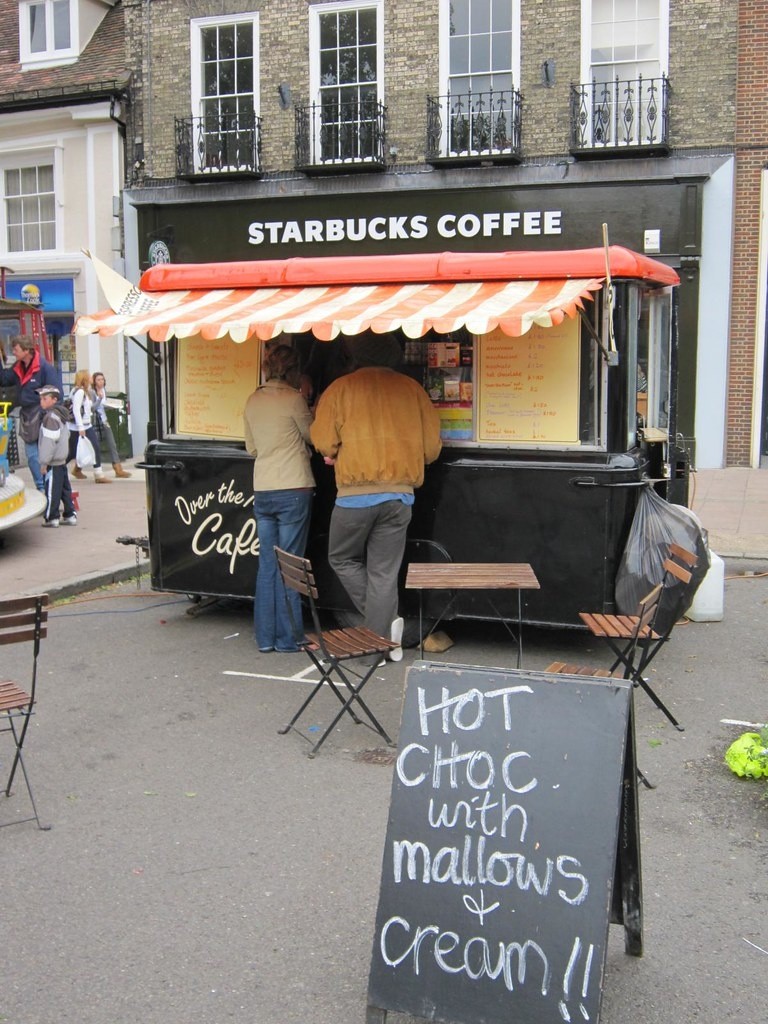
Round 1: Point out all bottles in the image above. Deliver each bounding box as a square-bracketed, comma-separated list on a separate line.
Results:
[404, 342, 428, 362]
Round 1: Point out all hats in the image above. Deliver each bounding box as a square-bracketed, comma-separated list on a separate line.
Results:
[34, 385, 60, 396]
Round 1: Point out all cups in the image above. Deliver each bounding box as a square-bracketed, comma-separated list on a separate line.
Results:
[437, 344, 447, 366]
[446, 346, 456, 366]
[429, 348, 437, 366]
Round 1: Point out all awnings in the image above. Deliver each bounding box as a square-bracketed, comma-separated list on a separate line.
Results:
[70, 279, 602, 341]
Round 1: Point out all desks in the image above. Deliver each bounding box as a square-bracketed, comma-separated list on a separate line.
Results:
[410, 556, 541, 669]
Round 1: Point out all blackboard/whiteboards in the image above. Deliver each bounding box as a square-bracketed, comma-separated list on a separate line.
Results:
[362, 665, 634, 1024]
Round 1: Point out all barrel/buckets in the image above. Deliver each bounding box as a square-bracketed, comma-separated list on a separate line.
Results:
[683, 548, 725, 623]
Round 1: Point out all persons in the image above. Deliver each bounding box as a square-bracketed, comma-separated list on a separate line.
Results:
[72, 372, 132, 479]
[66, 370, 112, 483]
[0, 335, 64, 513]
[310, 344, 442, 668]
[34, 384, 78, 528]
[265, 328, 403, 407]
[244, 344, 317, 652]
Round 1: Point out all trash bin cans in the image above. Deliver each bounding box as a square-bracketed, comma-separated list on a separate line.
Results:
[95, 390, 133, 463]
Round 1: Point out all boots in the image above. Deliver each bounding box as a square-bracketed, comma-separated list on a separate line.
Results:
[113, 463, 131, 478]
[71, 462, 87, 479]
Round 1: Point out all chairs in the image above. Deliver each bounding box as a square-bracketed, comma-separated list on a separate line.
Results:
[0, 593, 48, 833]
[577, 542, 702, 731]
[542, 581, 665, 791]
[273, 546, 399, 758]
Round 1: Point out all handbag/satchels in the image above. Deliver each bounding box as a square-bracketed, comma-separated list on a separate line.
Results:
[62, 388, 86, 423]
[18, 410, 41, 443]
[90, 406, 107, 442]
[76, 435, 96, 467]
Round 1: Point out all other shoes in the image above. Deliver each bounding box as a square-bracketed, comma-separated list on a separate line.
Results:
[368, 658, 387, 669]
[95, 478, 113, 483]
[59, 515, 77, 525]
[300, 643, 320, 652]
[389, 617, 404, 662]
[41, 518, 59, 528]
